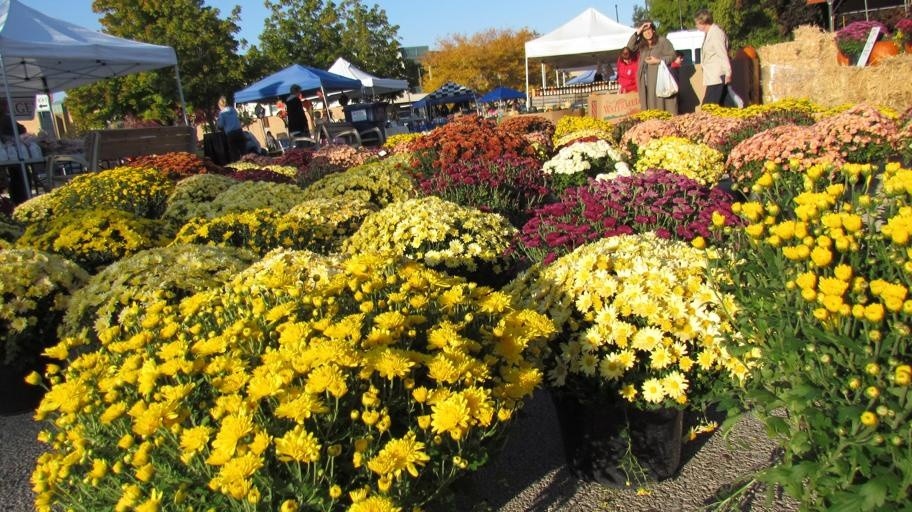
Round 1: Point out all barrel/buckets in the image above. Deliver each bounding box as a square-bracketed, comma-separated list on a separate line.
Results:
[0, 140, 43, 162]
[552, 391, 682, 489]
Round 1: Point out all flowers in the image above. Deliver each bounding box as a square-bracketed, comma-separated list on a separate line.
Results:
[1, 14, 912, 510]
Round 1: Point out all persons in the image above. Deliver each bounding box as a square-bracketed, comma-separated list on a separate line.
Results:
[593, 59, 610, 82]
[626, 22, 680, 117]
[286, 84, 312, 148]
[692, 10, 732, 108]
[615, 47, 642, 95]
[669, 51, 701, 115]
[511, 99, 520, 112]
[216, 95, 248, 162]
[600, 63, 617, 81]
[314, 109, 343, 140]
[0, 97, 34, 203]
[432, 101, 472, 120]
